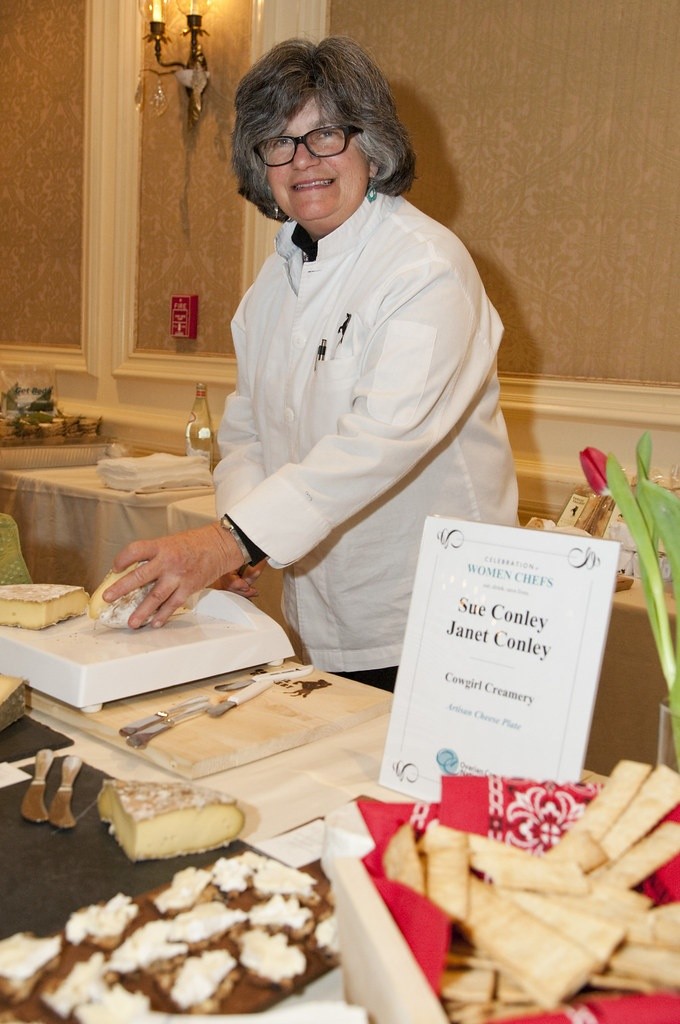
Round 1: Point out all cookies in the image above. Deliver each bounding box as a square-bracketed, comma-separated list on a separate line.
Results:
[0, 849, 343, 1024]
[381, 758, 680, 1024]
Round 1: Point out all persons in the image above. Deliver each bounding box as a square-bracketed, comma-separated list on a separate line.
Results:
[104, 38, 520, 697]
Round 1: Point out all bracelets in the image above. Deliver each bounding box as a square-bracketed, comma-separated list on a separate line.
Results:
[220, 516, 252, 564]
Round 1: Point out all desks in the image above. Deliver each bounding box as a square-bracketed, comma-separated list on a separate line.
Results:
[168, 491, 680, 775]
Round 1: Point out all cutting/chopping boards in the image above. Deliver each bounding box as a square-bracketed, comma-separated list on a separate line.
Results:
[26, 660, 393, 779]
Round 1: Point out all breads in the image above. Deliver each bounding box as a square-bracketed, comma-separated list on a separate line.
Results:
[0, 674, 26, 733]
[88, 560, 195, 629]
[98, 779, 246, 862]
[0, 584, 90, 629]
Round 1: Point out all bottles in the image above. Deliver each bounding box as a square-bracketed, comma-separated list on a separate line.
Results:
[185, 383, 213, 475]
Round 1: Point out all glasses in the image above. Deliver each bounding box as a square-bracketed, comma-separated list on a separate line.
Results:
[251, 123, 365, 168]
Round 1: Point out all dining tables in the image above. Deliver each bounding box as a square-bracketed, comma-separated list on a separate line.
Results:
[0, 433, 216, 597]
[0, 567, 680, 1024]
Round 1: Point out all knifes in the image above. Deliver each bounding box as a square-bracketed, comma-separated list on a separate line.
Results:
[48, 755, 82, 828]
[207, 678, 273, 716]
[215, 665, 313, 691]
[20, 749, 49, 822]
[120, 695, 208, 734]
[126, 702, 212, 749]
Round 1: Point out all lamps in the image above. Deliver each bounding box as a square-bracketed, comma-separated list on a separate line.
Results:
[134, 0, 216, 130]
